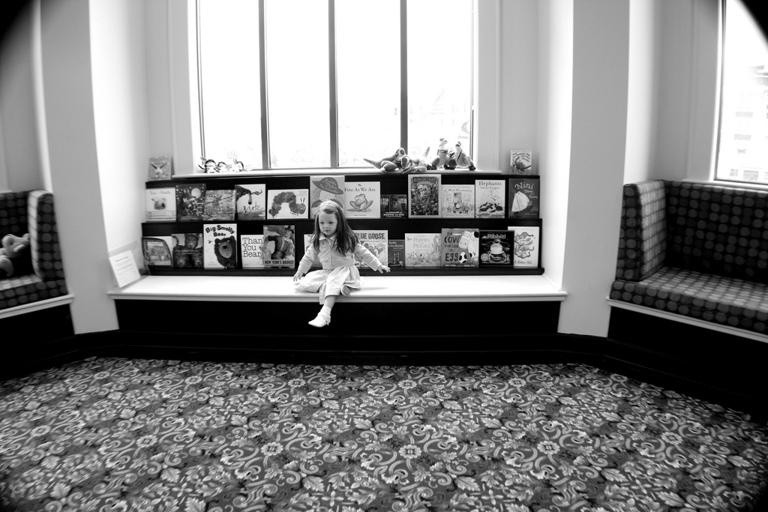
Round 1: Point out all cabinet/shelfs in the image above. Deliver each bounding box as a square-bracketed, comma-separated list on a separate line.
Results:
[141, 173, 545, 276]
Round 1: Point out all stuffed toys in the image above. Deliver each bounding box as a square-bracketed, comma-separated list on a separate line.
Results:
[363, 137, 476, 174]
[0, 233, 31, 276]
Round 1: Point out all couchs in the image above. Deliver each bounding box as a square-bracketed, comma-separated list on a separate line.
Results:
[0, 186, 69, 314]
[609, 178, 768, 341]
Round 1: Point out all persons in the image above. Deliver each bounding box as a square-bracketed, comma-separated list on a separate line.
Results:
[293, 200, 392, 328]
